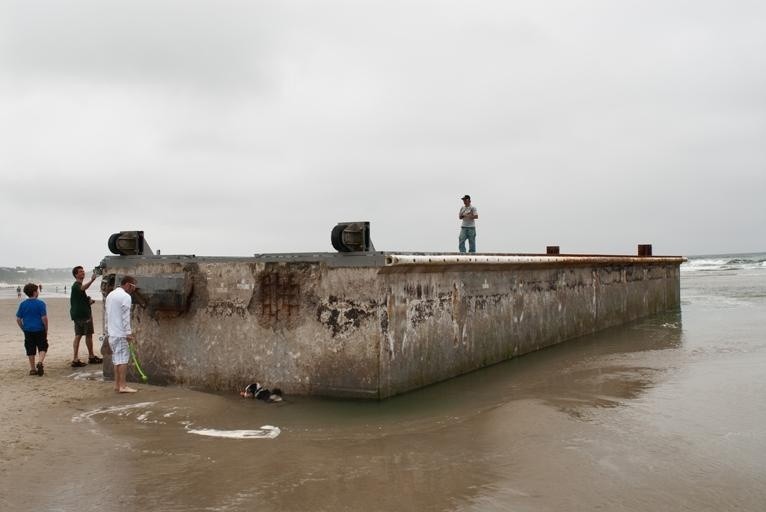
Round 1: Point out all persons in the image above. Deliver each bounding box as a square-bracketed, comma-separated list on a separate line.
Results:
[14, 283, 48, 375]
[16, 285, 21, 298]
[457, 194, 478, 252]
[38, 283, 42, 292]
[68, 266, 102, 367]
[101, 274, 139, 392]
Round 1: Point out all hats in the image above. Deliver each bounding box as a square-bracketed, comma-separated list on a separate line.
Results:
[461, 195, 470, 200]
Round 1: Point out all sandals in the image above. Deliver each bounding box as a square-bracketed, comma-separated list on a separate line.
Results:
[88, 356, 103, 364]
[71, 359, 86, 367]
[30, 362, 44, 376]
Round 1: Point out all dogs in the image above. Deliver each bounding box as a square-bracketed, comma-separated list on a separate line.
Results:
[240, 383, 284, 404]
